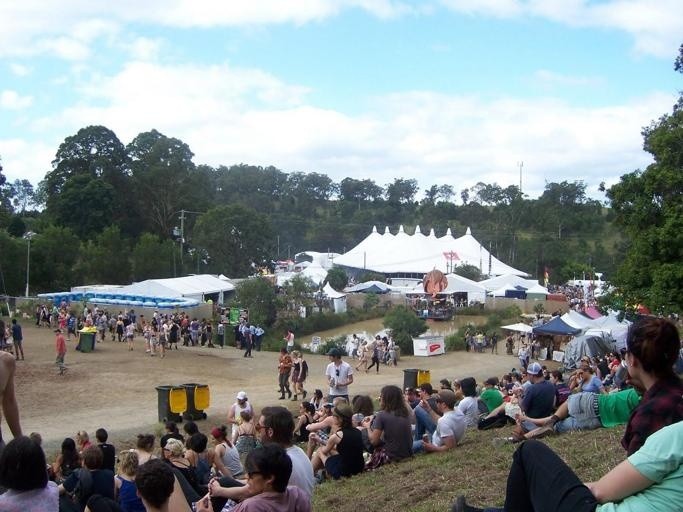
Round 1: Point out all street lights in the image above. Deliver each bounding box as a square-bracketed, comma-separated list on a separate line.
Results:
[23, 232, 37, 297]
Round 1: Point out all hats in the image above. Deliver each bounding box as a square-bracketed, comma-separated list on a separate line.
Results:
[522, 361, 544, 376]
[431, 389, 457, 404]
[325, 349, 341, 356]
[331, 404, 354, 419]
[237, 391, 248, 401]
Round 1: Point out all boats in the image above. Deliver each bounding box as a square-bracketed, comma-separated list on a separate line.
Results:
[416, 309, 452, 320]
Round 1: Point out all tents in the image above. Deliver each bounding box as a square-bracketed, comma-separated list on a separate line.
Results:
[499, 321, 534, 334]
[552, 307, 634, 353]
[532, 313, 582, 341]
[293, 261, 311, 270]
[300, 281, 347, 318]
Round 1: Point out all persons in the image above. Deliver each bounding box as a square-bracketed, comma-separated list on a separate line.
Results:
[278, 347, 293, 400]
[457, 378, 481, 431]
[11, 319, 25, 360]
[134, 457, 176, 512]
[172, 443, 313, 512]
[58, 444, 113, 512]
[163, 438, 194, 484]
[75, 430, 92, 453]
[309, 389, 325, 411]
[439, 378, 455, 393]
[479, 377, 504, 413]
[482, 362, 560, 425]
[413, 383, 437, 413]
[306, 396, 348, 459]
[53, 328, 70, 375]
[500, 374, 515, 396]
[352, 395, 373, 429]
[293, 401, 314, 443]
[361, 384, 417, 462]
[413, 389, 466, 452]
[296, 351, 308, 394]
[549, 369, 571, 410]
[185, 432, 233, 491]
[451, 420, 683, 512]
[577, 350, 621, 376]
[464, 325, 554, 368]
[282, 330, 295, 355]
[85, 451, 146, 512]
[206, 406, 314, 512]
[0, 435, 60, 512]
[406, 294, 467, 318]
[510, 372, 523, 403]
[209, 424, 244, 478]
[67, 296, 265, 359]
[52, 438, 81, 484]
[231, 409, 256, 455]
[292, 350, 308, 401]
[0, 319, 23, 457]
[227, 390, 255, 445]
[453, 380, 464, 401]
[325, 347, 354, 402]
[311, 403, 365, 480]
[160, 422, 184, 457]
[130, 433, 157, 465]
[3, 323, 14, 345]
[95, 428, 116, 476]
[576, 364, 607, 394]
[620, 318, 683, 457]
[35, 298, 67, 334]
[351, 334, 401, 373]
[491, 388, 642, 447]
[183, 422, 200, 435]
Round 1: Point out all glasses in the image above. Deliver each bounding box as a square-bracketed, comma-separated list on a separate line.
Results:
[246, 470, 267, 479]
[620, 347, 630, 359]
[254, 423, 270, 432]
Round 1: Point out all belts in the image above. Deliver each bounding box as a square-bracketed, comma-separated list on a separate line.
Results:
[593, 392, 604, 426]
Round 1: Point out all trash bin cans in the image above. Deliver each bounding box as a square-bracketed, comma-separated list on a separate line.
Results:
[76, 331, 97, 351]
[182, 383, 210, 421]
[402, 369, 431, 394]
[79, 332, 94, 352]
[155, 385, 188, 424]
